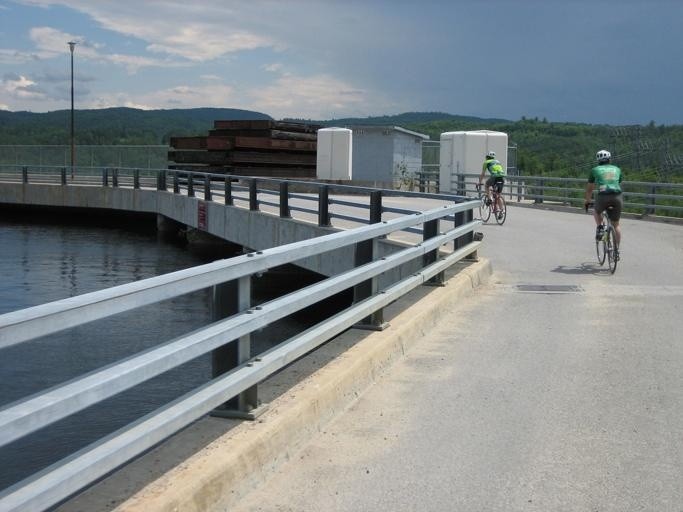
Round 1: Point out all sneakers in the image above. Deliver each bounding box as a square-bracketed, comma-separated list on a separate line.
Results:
[613, 246, 620, 262]
[498, 210, 505, 219]
[596, 224, 605, 243]
[486, 198, 493, 206]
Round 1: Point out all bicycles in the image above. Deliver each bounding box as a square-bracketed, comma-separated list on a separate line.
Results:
[585, 203, 621, 273]
[476, 182, 506, 225]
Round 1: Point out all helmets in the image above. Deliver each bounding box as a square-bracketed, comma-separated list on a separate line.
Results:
[596, 150, 611, 161]
[485, 152, 495, 159]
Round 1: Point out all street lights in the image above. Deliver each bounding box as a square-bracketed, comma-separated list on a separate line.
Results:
[67, 40, 80, 179]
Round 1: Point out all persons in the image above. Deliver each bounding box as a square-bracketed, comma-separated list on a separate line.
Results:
[476, 152, 506, 220]
[586, 150, 622, 262]
